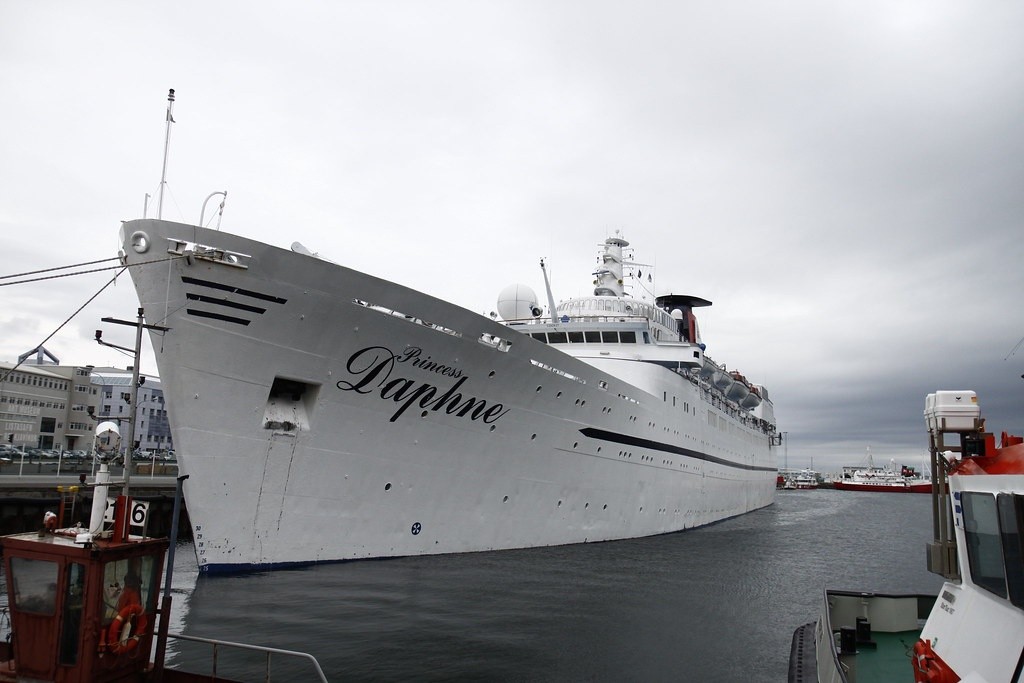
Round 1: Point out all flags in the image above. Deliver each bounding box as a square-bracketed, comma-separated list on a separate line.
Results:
[638, 270, 642, 278]
[648, 274, 651, 281]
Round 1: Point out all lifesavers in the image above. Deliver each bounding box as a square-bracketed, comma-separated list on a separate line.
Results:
[912, 642, 928, 682]
[108, 604, 148, 655]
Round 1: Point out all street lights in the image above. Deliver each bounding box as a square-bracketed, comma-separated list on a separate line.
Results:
[77, 368, 106, 457]
[131, 398, 158, 461]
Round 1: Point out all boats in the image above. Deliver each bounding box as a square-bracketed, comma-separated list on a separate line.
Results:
[691, 356, 718, 378]
[708, 365, 732, 389]
[777, 445, 949, 494]
[723, 369, 750, 402]
[123, 219, 786, 568]
[0, 414, 327, 683]
[739, 383, 762, 409]
[789, 389, 1024, 683]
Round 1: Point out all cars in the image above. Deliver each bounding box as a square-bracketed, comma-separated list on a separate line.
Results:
[0, 443, 176, 460]
